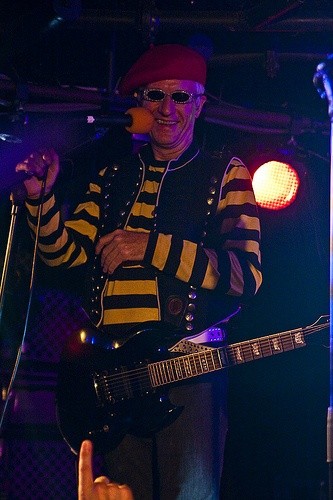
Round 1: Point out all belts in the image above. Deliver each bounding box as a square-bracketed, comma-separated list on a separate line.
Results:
[169, 327, 225, 355]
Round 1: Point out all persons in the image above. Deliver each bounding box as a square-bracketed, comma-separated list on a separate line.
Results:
[15, 45, 262, 500]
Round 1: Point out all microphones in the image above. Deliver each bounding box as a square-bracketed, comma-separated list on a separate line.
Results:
[85, 106, 154, 134]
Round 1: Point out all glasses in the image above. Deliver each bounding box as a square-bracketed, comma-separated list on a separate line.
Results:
[139, 88, 205, 104]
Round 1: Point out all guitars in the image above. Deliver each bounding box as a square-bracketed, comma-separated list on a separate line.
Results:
[55, 306, 330, 456]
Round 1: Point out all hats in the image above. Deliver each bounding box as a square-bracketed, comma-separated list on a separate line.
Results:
[120, 44, 207, 96]
